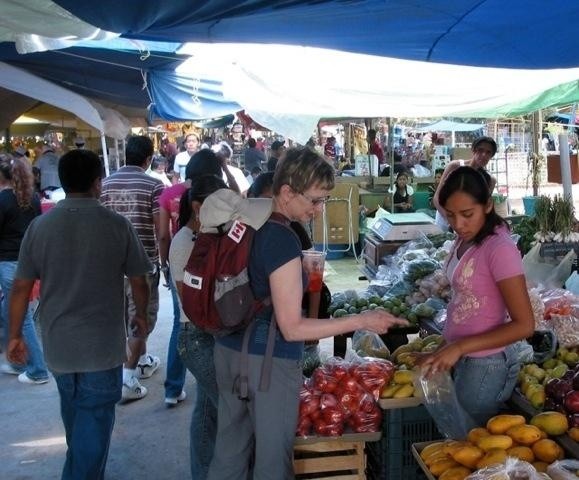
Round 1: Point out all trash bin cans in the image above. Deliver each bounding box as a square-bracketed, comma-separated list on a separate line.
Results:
[523, 196, 540, 216]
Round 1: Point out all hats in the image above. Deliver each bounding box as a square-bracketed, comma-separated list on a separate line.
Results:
[198, 189, 276, 234]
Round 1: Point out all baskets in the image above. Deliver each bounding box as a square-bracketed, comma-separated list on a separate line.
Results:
[369, 406, 444, 480]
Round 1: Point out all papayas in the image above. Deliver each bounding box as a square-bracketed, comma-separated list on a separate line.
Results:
[331, 296, 417, 328]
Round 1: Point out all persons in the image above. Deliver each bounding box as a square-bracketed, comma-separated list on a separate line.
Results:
[6, 149, 151, 479]
[1, 111, 578, 406]
[403, 164, 535, 434]
[204, 142, 409, 480]
[168, 177, 256, 479]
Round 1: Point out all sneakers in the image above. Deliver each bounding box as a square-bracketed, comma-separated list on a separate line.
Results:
[1, 363, 20, 375]
[164, 391, 186, 405]
[122, 375, 147, 399]
[17, 371, 49, 384]
[138, 354, 160, 379]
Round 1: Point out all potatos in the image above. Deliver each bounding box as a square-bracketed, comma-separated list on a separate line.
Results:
[406, 270, 451, 305]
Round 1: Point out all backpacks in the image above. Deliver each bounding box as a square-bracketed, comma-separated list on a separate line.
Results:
[181, 213, 302, 341]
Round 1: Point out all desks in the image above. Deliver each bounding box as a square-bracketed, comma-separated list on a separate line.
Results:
[311, 182, 361, 264]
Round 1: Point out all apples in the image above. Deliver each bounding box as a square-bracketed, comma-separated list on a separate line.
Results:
[298, 363, 392, 438]
[518, 347, 579, 432]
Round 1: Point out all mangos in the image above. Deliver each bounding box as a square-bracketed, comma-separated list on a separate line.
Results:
[383, 371, 417, 400]
[420, 412, 570, 479]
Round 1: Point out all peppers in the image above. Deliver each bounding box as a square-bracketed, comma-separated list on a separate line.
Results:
[510, 224, 535, 256]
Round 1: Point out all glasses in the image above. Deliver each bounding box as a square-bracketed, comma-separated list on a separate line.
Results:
[288, 183, 330, 207]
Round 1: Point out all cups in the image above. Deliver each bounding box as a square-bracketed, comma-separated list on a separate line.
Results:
[302, 249, 327, 293]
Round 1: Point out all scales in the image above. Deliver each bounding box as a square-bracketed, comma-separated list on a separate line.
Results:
[372, 212, 443, 243]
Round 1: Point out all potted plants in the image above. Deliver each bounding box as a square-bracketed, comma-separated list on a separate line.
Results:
[522, 149, 547, 218]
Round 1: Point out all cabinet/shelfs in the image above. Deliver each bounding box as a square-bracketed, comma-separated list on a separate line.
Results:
[359, 237, 408, 285]
[543, 149, 579, 186]
